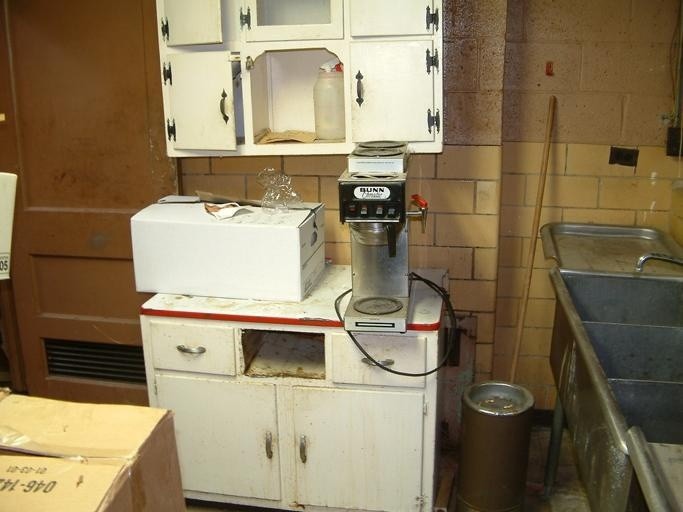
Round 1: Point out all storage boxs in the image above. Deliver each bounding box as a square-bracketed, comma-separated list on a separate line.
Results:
[0, 387, 188, 512]
[128, 189, 327, 301]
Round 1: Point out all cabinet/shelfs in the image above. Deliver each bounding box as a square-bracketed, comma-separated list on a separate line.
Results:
[152, 0, 445, 159]
[137, 264, 451, 512]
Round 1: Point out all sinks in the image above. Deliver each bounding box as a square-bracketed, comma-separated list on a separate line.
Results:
[577, 322, 683, 383]
[557, 271, 683, 327]
[605, 379, 683, 444]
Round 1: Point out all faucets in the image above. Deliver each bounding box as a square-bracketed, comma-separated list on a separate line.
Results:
[407, 192, 429, 232]
[635, 253, 683, 273]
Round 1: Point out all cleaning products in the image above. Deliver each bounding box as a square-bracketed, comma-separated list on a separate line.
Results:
[320, 57, 345, 71]
